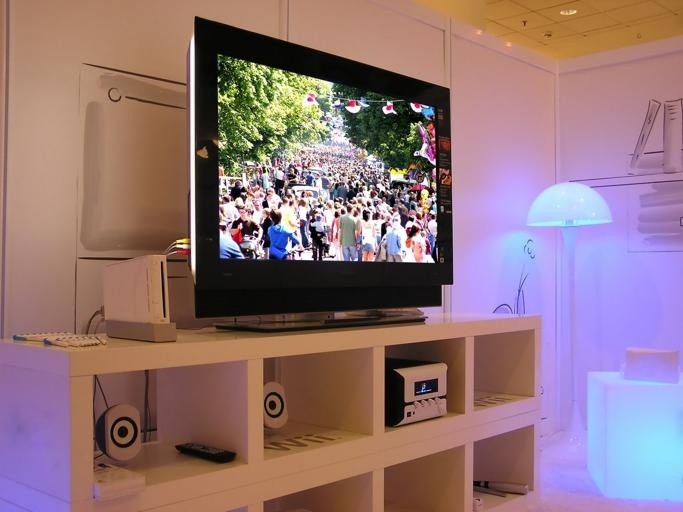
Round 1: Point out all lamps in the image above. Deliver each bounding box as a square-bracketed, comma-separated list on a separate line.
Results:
[524, 181, 614, 465]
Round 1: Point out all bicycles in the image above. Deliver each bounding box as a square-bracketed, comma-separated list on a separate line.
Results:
[287, 244, 314, 261]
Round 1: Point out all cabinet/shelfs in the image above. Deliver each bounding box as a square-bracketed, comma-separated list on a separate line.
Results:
[0, 313, 542, 512]
[569, 174, 683, 253]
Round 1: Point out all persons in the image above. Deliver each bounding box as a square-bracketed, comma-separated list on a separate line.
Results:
[218, 107, 438, 263]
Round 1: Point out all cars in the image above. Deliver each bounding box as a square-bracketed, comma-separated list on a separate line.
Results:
[288, 166, 332, 201]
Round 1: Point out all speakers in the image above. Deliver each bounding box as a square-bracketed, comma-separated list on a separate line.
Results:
[95, 405, 141, 461]
[263, 382, 288, 429]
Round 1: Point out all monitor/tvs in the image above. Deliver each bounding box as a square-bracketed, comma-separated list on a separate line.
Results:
[185, 14, 453, 333]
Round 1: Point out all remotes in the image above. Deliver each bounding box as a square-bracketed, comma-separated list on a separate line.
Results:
[175, 442, 236, 463]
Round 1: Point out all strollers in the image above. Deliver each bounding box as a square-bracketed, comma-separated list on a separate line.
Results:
[239, 224, 265, 259]
